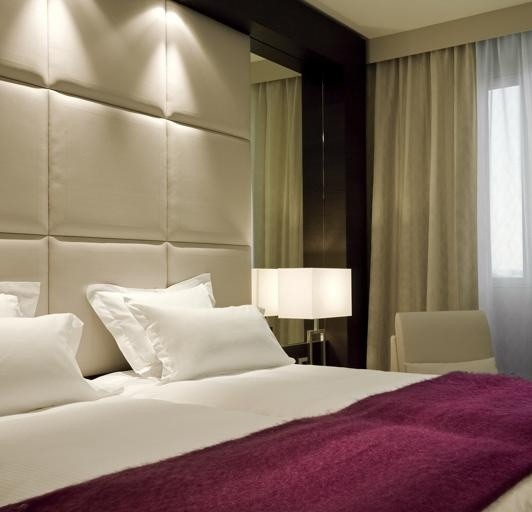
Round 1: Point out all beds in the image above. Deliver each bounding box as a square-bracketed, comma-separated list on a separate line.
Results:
[0, 363, 531, 512]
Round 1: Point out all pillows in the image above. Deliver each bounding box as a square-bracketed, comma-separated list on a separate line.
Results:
[1, 281, 41, 318]
[85, 273, 216, 378]
[132, 301, 295, 383]
[0, 312, 123, 416]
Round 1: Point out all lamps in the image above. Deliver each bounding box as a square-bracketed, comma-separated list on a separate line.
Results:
[278, 266, 352, 364]
[252, 268, 278, 318]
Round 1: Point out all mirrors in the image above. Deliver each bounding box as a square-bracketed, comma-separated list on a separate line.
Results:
[250, 50, 306, 348]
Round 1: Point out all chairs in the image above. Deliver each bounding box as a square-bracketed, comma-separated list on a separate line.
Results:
[390, 309, 498, 377]
